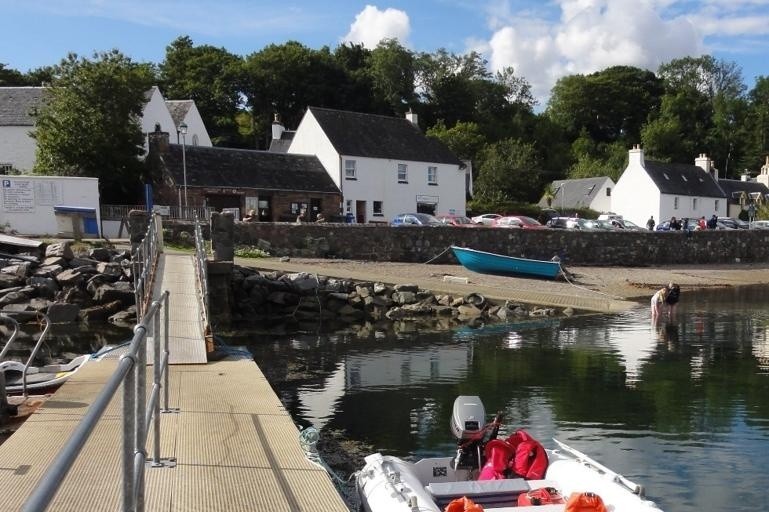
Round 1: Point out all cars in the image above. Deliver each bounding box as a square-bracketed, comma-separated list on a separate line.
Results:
[392, 212, 545, 229]
[549, 213, 646, 230]
[656, 217, 769, 230]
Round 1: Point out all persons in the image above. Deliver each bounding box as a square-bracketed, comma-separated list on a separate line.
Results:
[315, 213, 327, 221]
[669, 215, 677, 230]
[708, 214, 717, 228]
[241, 208, 258, 223]
[699, 215, 707, 230]
[649, 288, 671, 318]
[646, 214, 655, 231]
[665, 281, 681, 314]
[296, 208, 308, 224]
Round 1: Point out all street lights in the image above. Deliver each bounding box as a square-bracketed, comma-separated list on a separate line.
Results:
[177, 121, 188, 218]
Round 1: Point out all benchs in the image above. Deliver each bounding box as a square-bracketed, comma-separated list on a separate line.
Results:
[425, 476, 529, 504]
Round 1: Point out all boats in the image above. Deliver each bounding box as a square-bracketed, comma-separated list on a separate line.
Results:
[450, 246, 561, 278]
[355, 395, 661, 512]
[0, 310, 93, 396]
